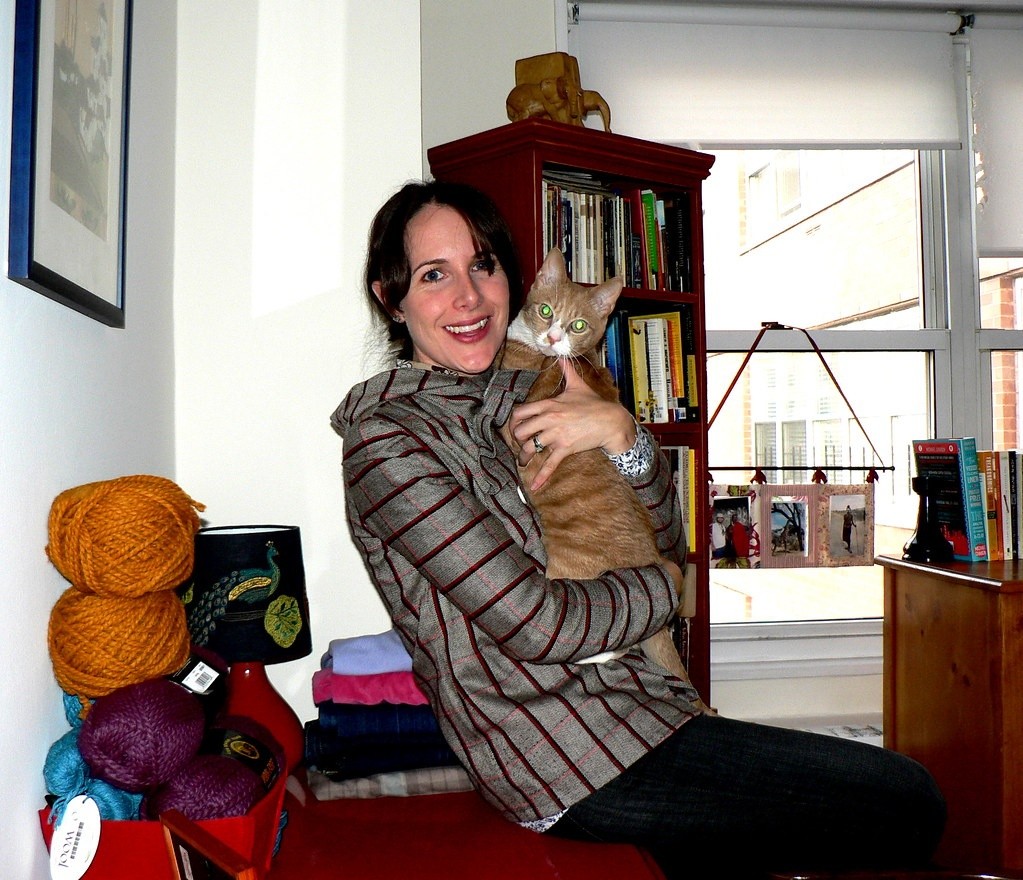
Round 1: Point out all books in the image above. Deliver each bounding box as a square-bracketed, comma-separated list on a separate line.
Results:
[541, 179, 695, 552]
[913, 437, 1023, 562]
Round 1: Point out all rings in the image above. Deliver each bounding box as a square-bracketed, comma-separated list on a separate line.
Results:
[532, 436, 546, 453]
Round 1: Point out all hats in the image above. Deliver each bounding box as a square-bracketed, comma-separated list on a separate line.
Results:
[716, 513, 723, 517]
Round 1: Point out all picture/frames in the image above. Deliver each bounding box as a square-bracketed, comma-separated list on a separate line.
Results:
[759, 484, 813, 568]
[819, 482, 875, 568]
[708, 484, 761, 568]
[8, 0, 133, 326]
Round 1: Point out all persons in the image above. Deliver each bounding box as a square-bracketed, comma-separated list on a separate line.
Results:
[842, 505, 857, 554]
[712, 512, 739, 559]
[329, 182, 949, 880]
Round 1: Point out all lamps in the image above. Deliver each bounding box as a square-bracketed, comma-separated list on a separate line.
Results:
[173, 524, 313, 776]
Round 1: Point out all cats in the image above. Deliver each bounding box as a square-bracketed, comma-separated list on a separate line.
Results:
[491, 246, 723, 718]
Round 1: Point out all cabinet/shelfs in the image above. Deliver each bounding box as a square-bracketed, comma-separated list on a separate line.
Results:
[427, 120, 716, 715]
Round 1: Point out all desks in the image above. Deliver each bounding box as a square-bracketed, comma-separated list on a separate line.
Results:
[875, 554, 1023, 880]
